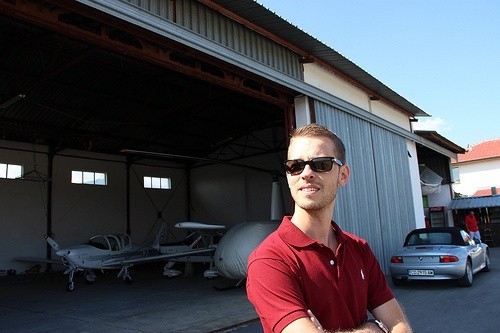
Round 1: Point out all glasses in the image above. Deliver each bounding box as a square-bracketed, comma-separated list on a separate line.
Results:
[282, 156, 343, 176]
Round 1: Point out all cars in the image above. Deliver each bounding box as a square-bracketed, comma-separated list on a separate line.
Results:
[389, 225, 491, 288]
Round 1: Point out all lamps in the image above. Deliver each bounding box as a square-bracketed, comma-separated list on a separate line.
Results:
[0, 93, 26, 109]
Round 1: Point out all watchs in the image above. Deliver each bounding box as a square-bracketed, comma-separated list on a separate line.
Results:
[368, 319, 389, 333]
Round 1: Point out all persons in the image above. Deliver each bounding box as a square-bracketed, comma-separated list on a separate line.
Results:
[465, 211, 481, 240]
[246, 123, 412, 333]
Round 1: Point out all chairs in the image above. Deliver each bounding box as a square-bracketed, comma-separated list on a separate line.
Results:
[440, 236, 451, 243]
[413, 237, 425, 244]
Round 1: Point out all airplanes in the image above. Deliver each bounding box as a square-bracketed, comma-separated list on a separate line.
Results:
[13, 221, 227, 293]
